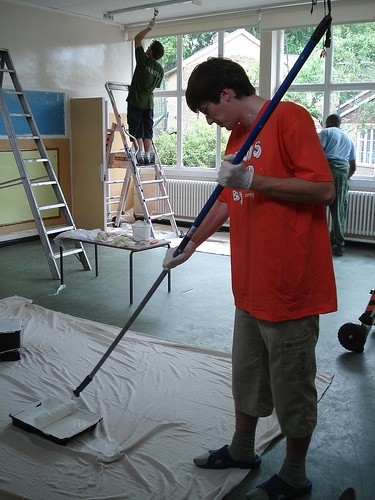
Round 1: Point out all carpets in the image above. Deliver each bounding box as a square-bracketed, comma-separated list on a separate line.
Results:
[120, 219, 230, 255]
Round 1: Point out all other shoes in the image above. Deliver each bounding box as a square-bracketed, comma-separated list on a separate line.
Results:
[333, 247, 345, 256]
[144, 157, 151, 165]
[137, 157, 144, 164]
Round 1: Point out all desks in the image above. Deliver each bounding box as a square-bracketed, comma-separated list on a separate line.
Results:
[53, 228, 172, 304]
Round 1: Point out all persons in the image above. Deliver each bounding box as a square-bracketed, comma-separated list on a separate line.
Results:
[318, 114, 357, 256]
[163, 55, 338, 500]
[126, 18, 164, 166]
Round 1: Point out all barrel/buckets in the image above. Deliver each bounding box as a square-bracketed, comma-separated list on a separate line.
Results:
[131, 224, 152, 241]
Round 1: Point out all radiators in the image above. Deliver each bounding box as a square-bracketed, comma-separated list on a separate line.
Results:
[154, 178, 375, 243]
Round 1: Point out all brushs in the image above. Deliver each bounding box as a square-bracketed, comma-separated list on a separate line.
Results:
[36, 13, 333, 429]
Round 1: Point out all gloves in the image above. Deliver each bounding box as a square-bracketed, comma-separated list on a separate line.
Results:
[216, 153, 253, 191]
[149, 18, 156, 29]
[163, 240, 196, 270]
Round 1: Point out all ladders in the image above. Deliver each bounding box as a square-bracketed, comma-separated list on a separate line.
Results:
[104, 81, 181, 243]
[0, 47, 92, 280]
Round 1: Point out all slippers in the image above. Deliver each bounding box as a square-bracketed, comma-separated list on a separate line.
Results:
[255, 474, 312, 500]
[198, 444, 261, 469]
